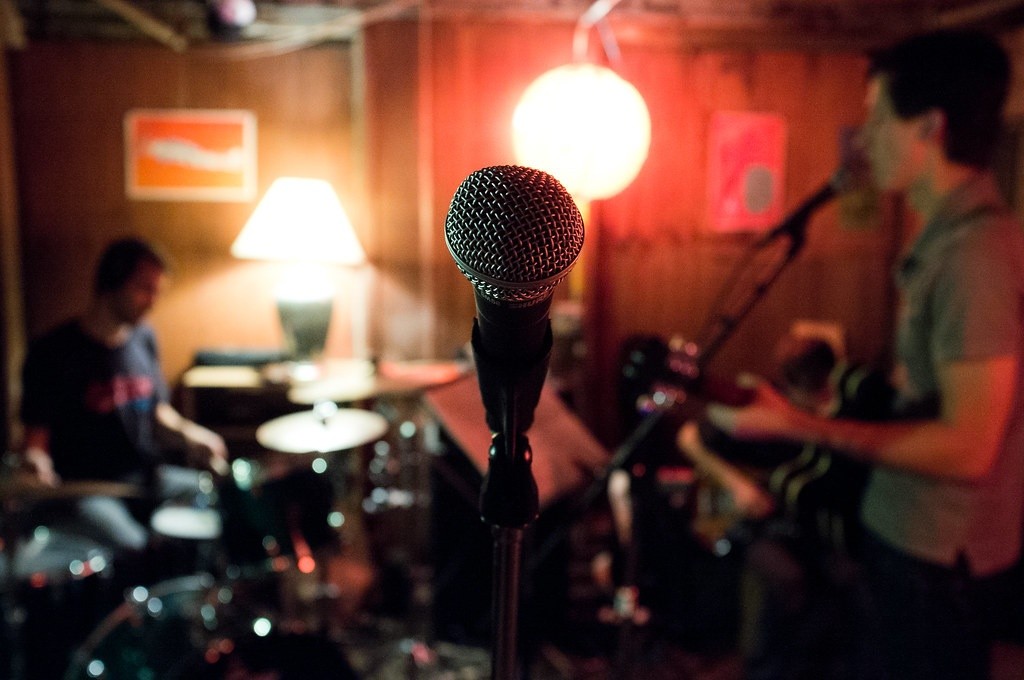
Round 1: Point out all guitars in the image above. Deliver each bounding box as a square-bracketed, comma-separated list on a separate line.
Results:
[610, 328, 898, 543]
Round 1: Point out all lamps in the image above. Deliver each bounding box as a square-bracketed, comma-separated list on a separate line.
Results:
[231, 177, 364, 360]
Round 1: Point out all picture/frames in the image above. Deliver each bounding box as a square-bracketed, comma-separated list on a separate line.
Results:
[123, 108, 258, 201]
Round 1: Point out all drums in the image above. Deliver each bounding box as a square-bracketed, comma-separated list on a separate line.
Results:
[63, 570, 281, 680]
[147, 507, 222, 583]
[11, 529, 127, 643]
[213, 463, 341, 571]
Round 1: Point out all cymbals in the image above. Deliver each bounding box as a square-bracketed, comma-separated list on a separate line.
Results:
[252, 405, 388, 455]
[285, 358, 475, 407]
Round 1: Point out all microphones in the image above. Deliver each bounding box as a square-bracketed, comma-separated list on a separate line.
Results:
[444, 163, 585, 535]
[759, 164, 860, 247]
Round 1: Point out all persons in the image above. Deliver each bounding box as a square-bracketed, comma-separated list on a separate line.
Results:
[22, 237, 227, 521]
[676, 25, 1023, 680]
